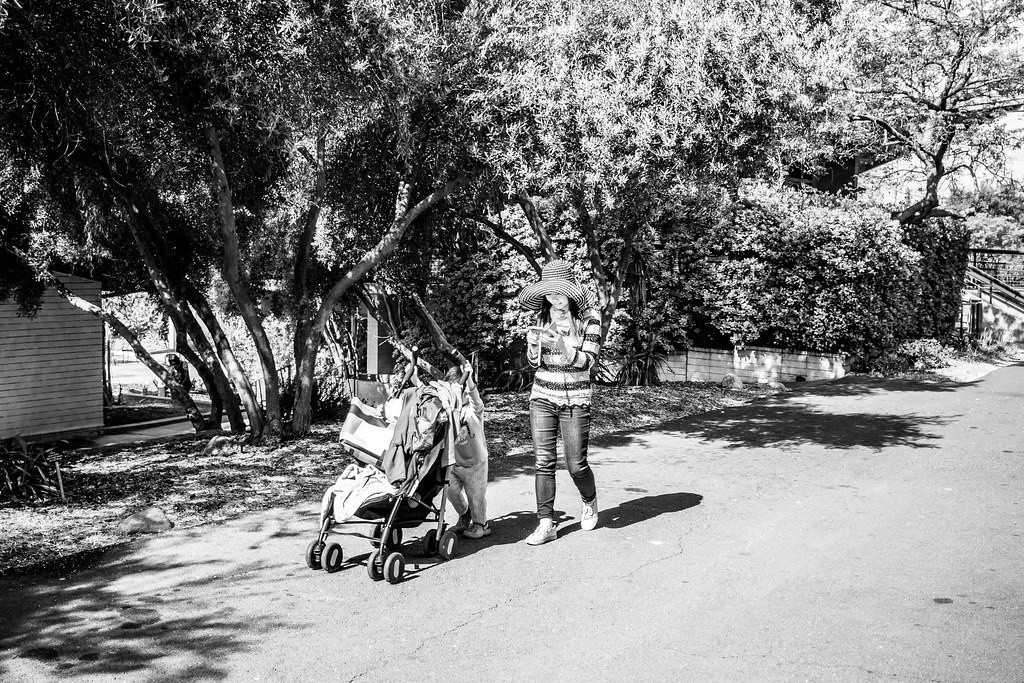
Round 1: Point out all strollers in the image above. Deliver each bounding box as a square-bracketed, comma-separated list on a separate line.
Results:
[305, 344, 470, 584]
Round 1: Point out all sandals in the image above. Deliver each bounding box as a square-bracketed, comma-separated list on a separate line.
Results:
[455, 505, 472, 531]
[463, 522, 491, 538]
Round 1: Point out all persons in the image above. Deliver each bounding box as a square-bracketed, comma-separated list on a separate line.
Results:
[164, 353, 192, 398]
[519, 261, 602, 545]
[406, 360, 491, 538]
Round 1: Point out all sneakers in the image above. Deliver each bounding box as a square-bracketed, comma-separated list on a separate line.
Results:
[525, 523, 558, 545]
[581, 498, 599, 530]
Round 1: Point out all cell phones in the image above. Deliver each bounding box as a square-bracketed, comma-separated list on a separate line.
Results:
[530, 321, 558, 337]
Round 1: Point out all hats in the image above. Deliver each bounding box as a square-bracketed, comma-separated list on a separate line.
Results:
[518, 259, 596, 314]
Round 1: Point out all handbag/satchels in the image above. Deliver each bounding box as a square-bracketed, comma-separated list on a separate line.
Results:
[339, 397, 399, 471]
